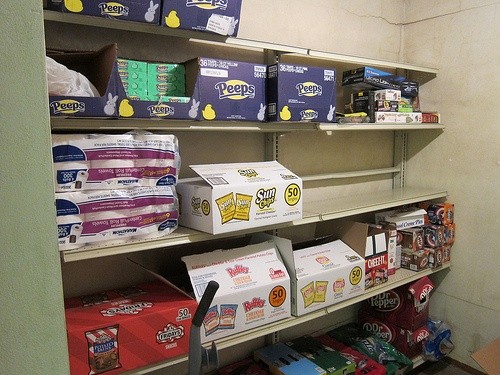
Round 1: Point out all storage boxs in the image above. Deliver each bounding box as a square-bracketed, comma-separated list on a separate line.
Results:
[49, 0, 455, 375]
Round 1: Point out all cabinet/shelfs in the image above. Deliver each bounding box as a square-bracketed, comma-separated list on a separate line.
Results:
[43, 9, 451, 375]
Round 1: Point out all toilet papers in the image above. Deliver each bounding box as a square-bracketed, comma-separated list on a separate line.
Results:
[52, 135, 181, 192]
[55, 186, 178, 251]
